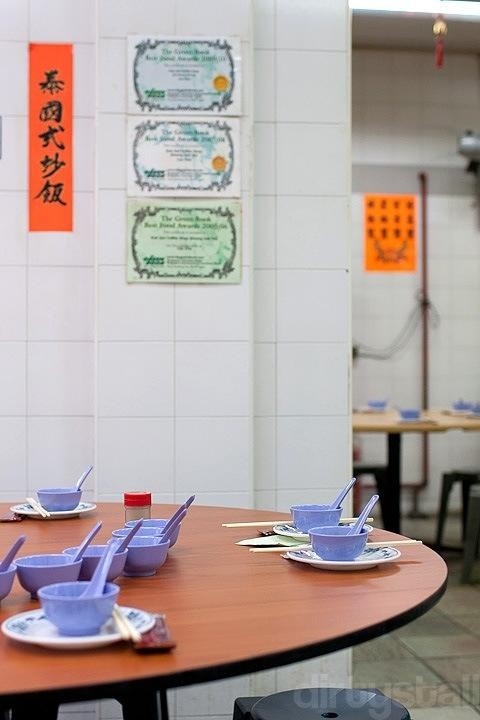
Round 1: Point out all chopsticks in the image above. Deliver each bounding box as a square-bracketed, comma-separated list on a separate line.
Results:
[222, 516, 373, 528]
[249, 538, 423, 553]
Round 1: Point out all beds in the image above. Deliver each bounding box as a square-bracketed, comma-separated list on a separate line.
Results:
[1, 496, 449, 719]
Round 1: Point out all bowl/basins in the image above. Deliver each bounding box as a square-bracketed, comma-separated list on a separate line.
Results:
[366, 395, 479, 420]
[0, 490, 371, 634]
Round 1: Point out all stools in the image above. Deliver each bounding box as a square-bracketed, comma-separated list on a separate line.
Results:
[453, 487, 479, 587]
[230, 684, 412, 720]
[433, 466, 479, 550]
[350, 457, 389, 535]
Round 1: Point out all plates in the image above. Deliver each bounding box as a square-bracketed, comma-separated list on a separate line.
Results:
[3, 602, 155, 652]
[273, 523, 373, 538]
[287, 542, 401, 571]
